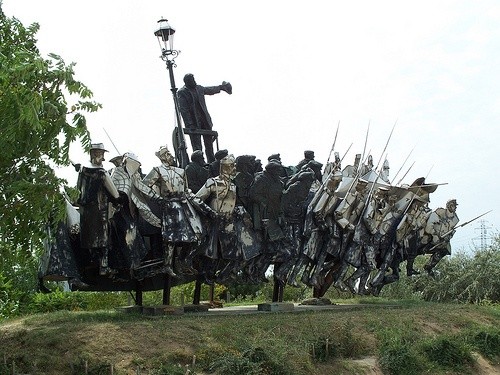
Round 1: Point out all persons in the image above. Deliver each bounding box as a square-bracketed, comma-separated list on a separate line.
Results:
[69, 72, 459, 299]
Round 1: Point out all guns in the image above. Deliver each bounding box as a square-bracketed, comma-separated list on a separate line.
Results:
[304, 118, 493, 254]
[101, 128, 120, 156]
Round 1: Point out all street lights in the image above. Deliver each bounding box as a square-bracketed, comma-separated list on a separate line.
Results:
[154, 16, 190, 170]
